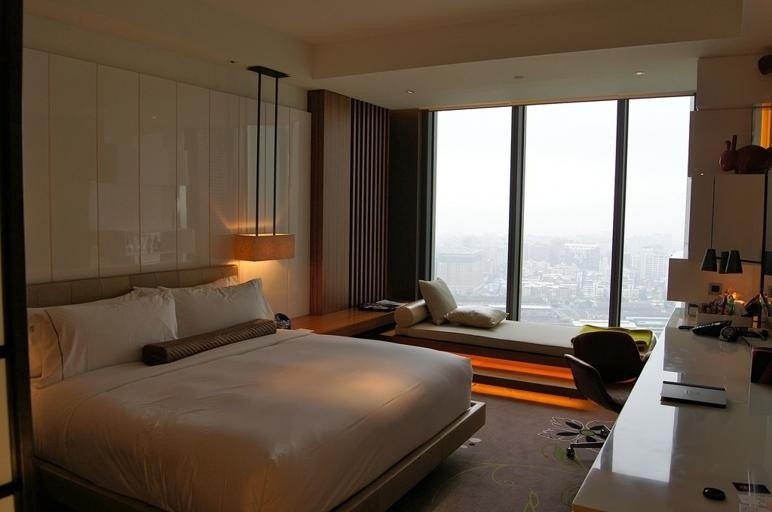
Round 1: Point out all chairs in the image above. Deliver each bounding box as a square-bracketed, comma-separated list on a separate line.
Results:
[564, 330, 644, 458]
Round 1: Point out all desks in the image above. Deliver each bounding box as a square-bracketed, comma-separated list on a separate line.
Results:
[572, 306, 772, 512]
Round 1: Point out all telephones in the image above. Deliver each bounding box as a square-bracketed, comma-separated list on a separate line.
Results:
[692, 319, 733, 337]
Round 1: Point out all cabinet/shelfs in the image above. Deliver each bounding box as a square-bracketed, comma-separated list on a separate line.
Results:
[666, 52, 772, 312]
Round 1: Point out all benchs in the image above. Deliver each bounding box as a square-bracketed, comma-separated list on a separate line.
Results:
[288, 299, 410, 335]
[395, 299, 596, 405]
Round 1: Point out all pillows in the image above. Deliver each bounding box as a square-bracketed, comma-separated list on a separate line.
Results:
[418, 277, 456, 325]
[135, 274, 241, 298]
[142, 316, 278, 367]
[444, 303, 509, 329]
[33, 287, 180, 389]
[159, 278, 275, 333]
[27, 289, 139, 380]
[394, 297, 428, 327]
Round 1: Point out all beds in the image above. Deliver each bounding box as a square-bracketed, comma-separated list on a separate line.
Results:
[26, 263, 486, 511]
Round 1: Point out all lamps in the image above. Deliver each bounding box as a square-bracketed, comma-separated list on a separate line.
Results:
[700, 248, 772, 277]
[233, 65, 297, 262]
